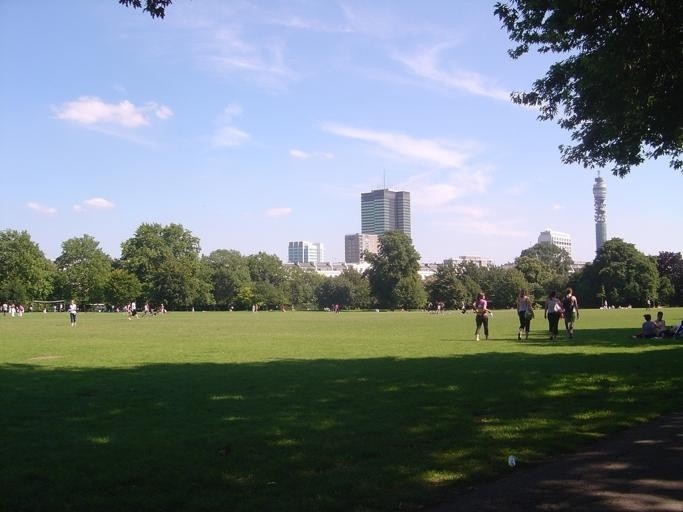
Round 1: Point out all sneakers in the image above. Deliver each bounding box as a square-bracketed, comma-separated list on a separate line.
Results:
[517, 332, 522, 340]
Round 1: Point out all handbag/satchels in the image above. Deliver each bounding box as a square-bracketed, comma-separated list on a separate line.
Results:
[525, 311, 534, 319]
[553, 303, 562, 313]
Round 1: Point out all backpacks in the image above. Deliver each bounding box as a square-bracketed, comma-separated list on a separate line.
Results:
[563, 295, 574, 311]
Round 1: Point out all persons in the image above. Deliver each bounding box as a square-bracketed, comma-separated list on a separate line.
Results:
[472, 298, 477, 313]
[330, 303, 341, 314]
[561, 288, 579, 340]
[516, 288, 535, 340]
[255, 303, 258, 312]
[252, 304, 256, 312]
[460, 297, 466, 315]
[474, 292, 491, 341]
[544, 290, 563, 339]
[600, 298, 683, 344]
[278, 302, 294, 313]
[106, 301, 167, 320]
[424, 300, 445, 315]
[0, 300, 78, 327]
